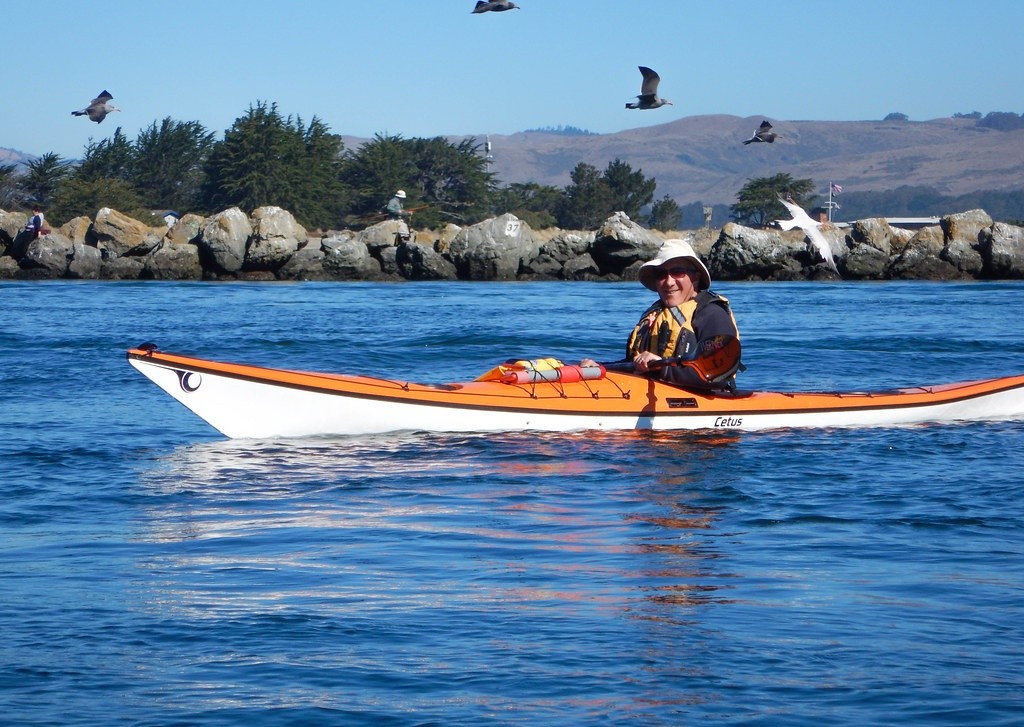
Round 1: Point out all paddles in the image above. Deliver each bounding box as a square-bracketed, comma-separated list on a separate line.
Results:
[504, 333, 743, 387]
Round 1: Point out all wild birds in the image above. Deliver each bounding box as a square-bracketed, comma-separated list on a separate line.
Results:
[470, 0, 520, 14]
[742, 120, 783, 145]
[71, 89, 122, 124]
[773, 190, 843, 280]
[625, 66, 674, 111]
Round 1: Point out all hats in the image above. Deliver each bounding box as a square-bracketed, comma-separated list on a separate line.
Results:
[638, 239, 711, 292]
[395, 190, 407, 199]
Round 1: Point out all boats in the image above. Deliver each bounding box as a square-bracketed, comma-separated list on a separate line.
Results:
[128, 342, 1024, 439]
[846, 217, 941, 230]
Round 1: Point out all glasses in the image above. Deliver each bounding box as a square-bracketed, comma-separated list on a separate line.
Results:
[654, 267, 699, 280]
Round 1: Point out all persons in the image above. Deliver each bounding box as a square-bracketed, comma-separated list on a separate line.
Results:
[624, 238, 745, 396]
[381, 189, 413, 221]
[0, 202, 44, 262]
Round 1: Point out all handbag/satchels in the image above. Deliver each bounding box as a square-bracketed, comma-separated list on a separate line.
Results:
[379, 206, 388, 214]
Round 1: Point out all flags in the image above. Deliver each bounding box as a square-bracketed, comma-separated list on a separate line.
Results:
[831, 183, 842, 197]
